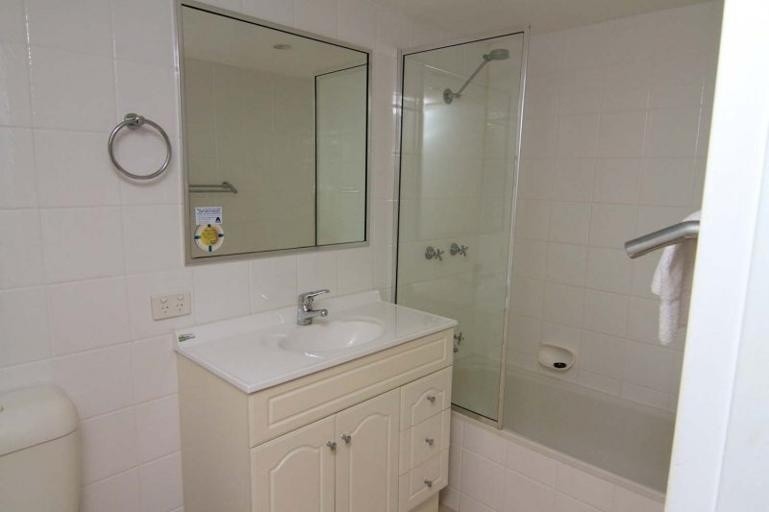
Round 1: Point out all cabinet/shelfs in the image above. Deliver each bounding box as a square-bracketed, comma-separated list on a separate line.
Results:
[178, 357, 398, 512]
[398, 365, 454, 510]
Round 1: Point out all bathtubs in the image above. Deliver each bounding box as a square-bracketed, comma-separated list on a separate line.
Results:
[439, 353, 676, 511]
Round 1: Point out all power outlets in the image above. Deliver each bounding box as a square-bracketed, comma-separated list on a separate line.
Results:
[150, 291, 191, 321]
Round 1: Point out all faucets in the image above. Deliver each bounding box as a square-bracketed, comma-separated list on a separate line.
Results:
[293, 287, 332, 325]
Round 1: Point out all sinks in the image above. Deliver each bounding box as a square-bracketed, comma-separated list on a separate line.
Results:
[281, 317, 387, 359]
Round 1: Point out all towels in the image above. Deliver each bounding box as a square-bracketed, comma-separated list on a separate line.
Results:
[649, 208, 702, 349]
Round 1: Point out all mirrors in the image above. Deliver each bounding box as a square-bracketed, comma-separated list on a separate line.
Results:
[172, 2, 374, 267]
